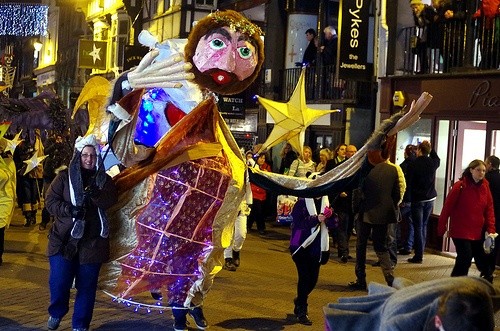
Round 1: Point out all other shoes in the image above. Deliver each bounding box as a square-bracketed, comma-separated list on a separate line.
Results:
[257, 229, 264, 234]
[39, 222, 46, 231]
[293, 297, 313, 325]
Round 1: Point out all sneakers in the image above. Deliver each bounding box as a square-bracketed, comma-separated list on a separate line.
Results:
[172, 320, 189, 331]
[47, 307, 69, 330]
[190, 306, 209, 329]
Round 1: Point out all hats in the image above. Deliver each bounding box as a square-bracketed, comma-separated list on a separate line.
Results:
[291, 172, 331, 264]
[71, 135, 109, 240]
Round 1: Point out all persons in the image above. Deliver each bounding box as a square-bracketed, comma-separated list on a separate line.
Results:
[343, 146, 397, 291]
[318, 25, 338, 72]
[43, 134, 115, 331]
[170, 301, 210, 330]
[409, 0, 500, 74]
[479, 155, 500, 272]
[295, 28, 319, 82]
[432, 282, 495, 330]
[289, 172, 335, 326]
[223, 131, 440, 272]
[0, 127, 61, 266]
[437, 159, 496, 279]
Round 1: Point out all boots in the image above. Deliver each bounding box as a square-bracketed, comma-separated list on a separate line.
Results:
[348, 274, 366, 291]
[232, 250, 240, 267]
[225, 258, 236, 271]
[384, 273, 394, 287]
[22, 209, 37, 226]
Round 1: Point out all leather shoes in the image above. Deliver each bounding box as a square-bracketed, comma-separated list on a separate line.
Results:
[340, 228, 423, 270]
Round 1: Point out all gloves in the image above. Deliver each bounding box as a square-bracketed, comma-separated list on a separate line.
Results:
[483, 231, 498, 255]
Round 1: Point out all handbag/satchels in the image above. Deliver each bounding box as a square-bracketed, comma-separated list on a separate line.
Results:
[277, 195, 296, 216]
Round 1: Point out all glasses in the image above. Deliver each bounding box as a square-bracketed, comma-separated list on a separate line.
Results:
[80, 154, 98, 159]
